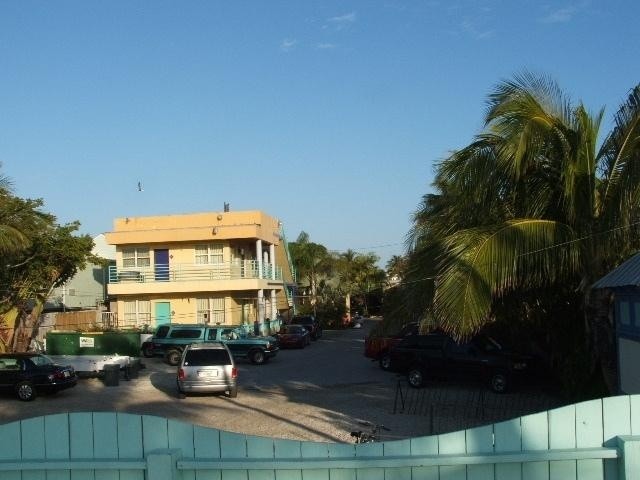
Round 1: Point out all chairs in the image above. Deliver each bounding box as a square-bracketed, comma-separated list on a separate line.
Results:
[0, 361, 6, 368]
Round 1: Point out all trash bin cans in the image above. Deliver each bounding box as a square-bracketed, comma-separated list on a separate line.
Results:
[104, 363, 121, 386]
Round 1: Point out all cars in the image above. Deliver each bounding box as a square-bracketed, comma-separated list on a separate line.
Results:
[292, 315, 323, 342]
[321, 300, 364, 331]
[388, 321, 536, 394]
[176, 341, 238, 399]
[0, 353, 79, 401]
[279, 324, 311, 349]
[365, 322, 443, 371]
[141, 325, 279, 366]
